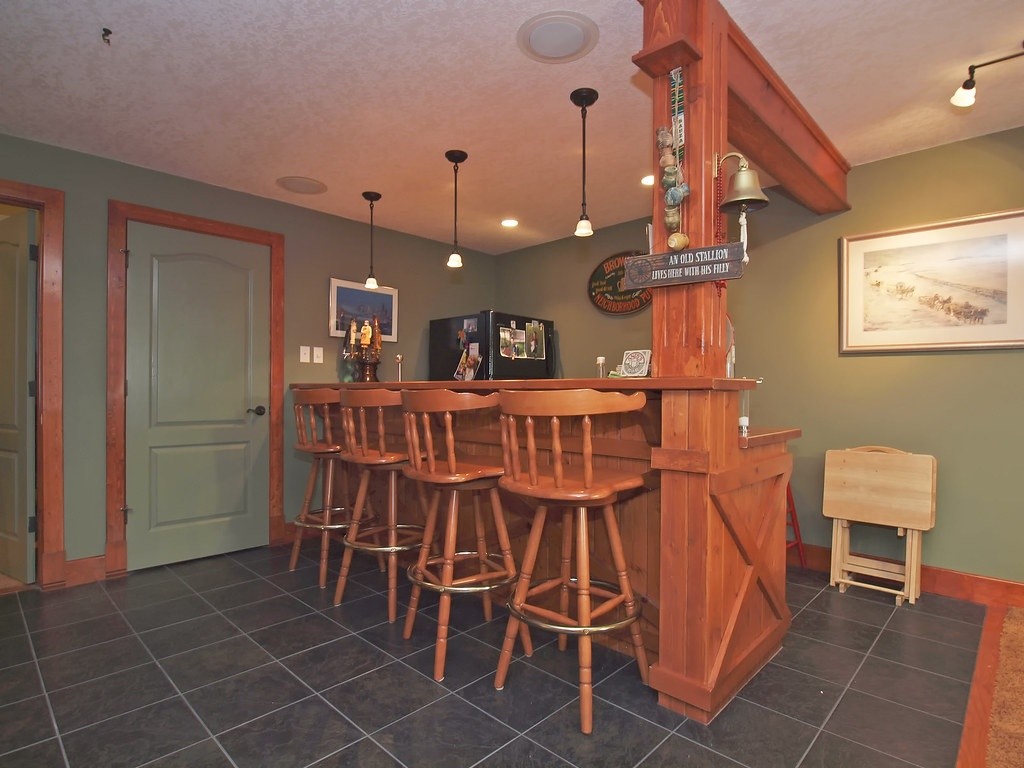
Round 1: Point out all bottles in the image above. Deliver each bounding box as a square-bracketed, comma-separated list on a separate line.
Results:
[593, 356, 608, 378]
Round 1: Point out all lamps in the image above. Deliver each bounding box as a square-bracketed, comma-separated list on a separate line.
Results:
[362, 191, 381, 289]
[570, 87, 599, 237]
[445, 150, 468, 268]
[949, 52, 1024, 107]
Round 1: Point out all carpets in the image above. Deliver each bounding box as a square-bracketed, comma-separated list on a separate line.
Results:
[985, 605, 1024, 768]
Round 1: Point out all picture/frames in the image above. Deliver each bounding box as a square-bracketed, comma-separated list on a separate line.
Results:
[838, 209, 1024, 356]
[329, 277, 398, 344]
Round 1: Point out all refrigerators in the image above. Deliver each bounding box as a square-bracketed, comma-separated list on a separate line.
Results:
[428, 309, 556, 380]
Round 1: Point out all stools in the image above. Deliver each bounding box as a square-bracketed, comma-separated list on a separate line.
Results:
[399, 387, 531, 682]
[333, 389, 437, 626]
[786, 481, 808, 570]
[495, 389, 649, 735]
[289, 387, 386, 590]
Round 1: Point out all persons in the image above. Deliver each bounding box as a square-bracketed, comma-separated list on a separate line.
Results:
[510, 337, 515, 351]
[457, 355, 478, 381]
[530, 331, 538, 357]
